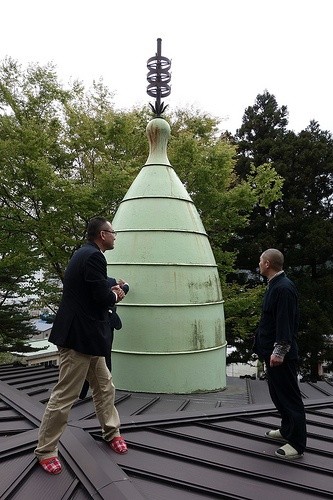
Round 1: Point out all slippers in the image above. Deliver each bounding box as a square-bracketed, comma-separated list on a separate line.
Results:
[265, 429, 289, 442]
[275, 443, 301, 459]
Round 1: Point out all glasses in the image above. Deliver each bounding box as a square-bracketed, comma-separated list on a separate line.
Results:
[99, 230, 117, 237]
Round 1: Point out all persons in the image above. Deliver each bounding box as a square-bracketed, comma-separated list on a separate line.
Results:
[33, 216, 127, 475]
[250, 249, 307, 460]
[75, 276, 129, 398]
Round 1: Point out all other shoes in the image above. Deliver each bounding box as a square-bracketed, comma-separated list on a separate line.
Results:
[41, 457, 63, 475]
[110, 436, 127, 455]
